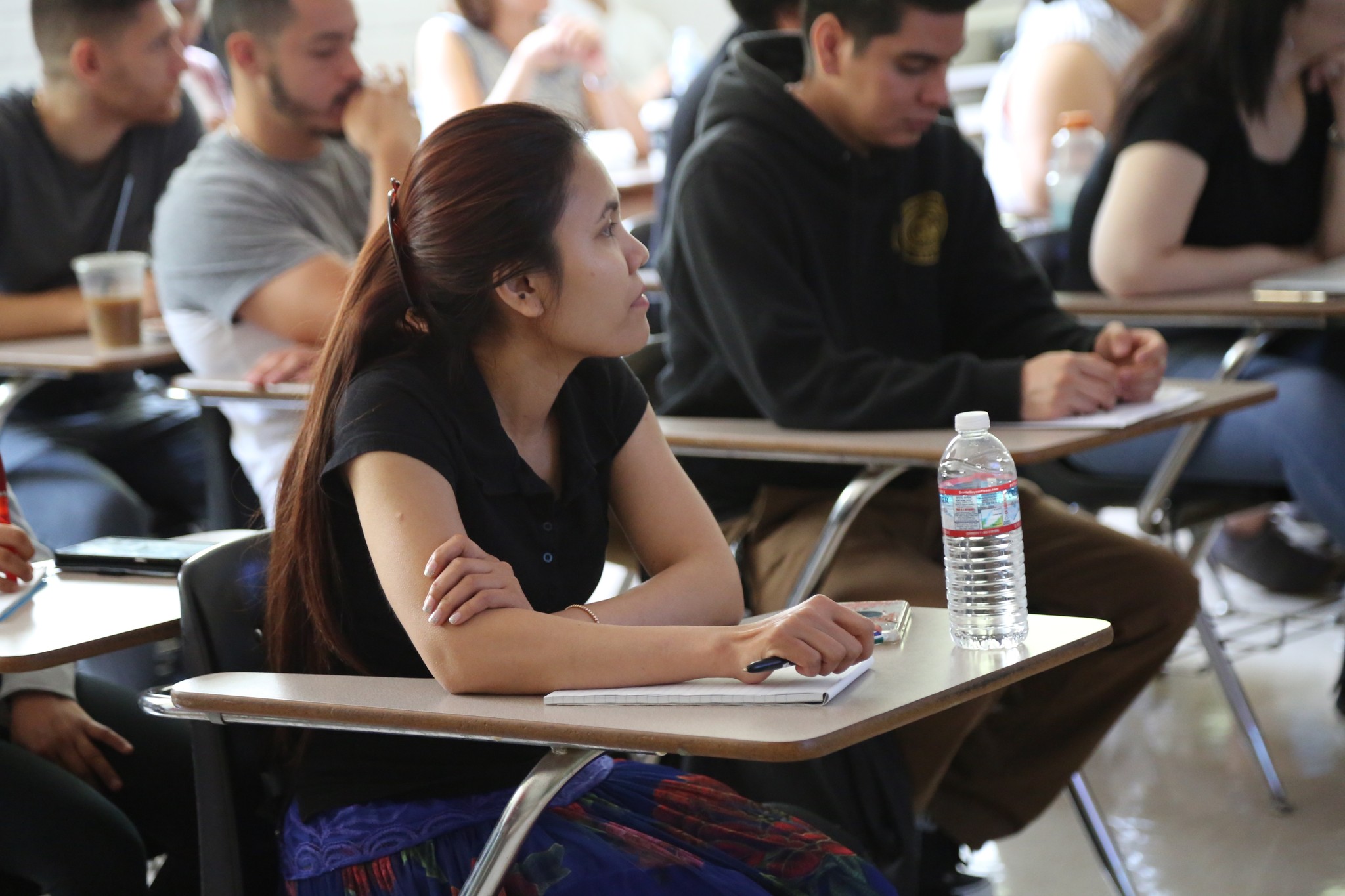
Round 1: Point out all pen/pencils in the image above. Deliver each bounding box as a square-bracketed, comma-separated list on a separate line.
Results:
[0, 454, 18, 584]
[741, 629, 883, 674]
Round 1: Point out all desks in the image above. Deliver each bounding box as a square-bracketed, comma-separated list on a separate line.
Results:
[0, 215, 1344, 895]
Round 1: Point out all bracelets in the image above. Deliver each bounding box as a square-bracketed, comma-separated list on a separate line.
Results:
[565, 605, 599, 624]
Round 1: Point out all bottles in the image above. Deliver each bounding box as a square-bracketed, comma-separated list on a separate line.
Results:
[1047, 109, 1104, 229]
[936, 411, 1028, 650]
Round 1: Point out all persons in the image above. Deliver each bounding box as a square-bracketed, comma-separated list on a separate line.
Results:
[151, 0, 421, 530]
[264, 102, 889, 896]
[0, 524, 201, 896]
[653, 0, 1199, 896]
[657, 0, 807, 247]
[985, 0, 1184, 238]
[411, 0, 650, 163]
[1057, 0, 1345, 603]
[0, 0, 233, 694]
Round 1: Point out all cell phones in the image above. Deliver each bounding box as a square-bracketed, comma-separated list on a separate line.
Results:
[53, 533, 219, 573]
[834, 600, 913, 642]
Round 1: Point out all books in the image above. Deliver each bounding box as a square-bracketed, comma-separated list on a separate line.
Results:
[0, 567, 49, 621]
[1253, 256, 1345, 297]
[544, 656, 868, 707]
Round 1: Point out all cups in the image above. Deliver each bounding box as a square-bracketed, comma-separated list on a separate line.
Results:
[71, 252, 148, 346]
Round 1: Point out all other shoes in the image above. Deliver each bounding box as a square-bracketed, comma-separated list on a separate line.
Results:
[1209, 519, 1342, 596]
[950, 873, 993, 896]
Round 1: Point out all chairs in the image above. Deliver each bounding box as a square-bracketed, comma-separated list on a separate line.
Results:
[176, 529, 377, 895]
